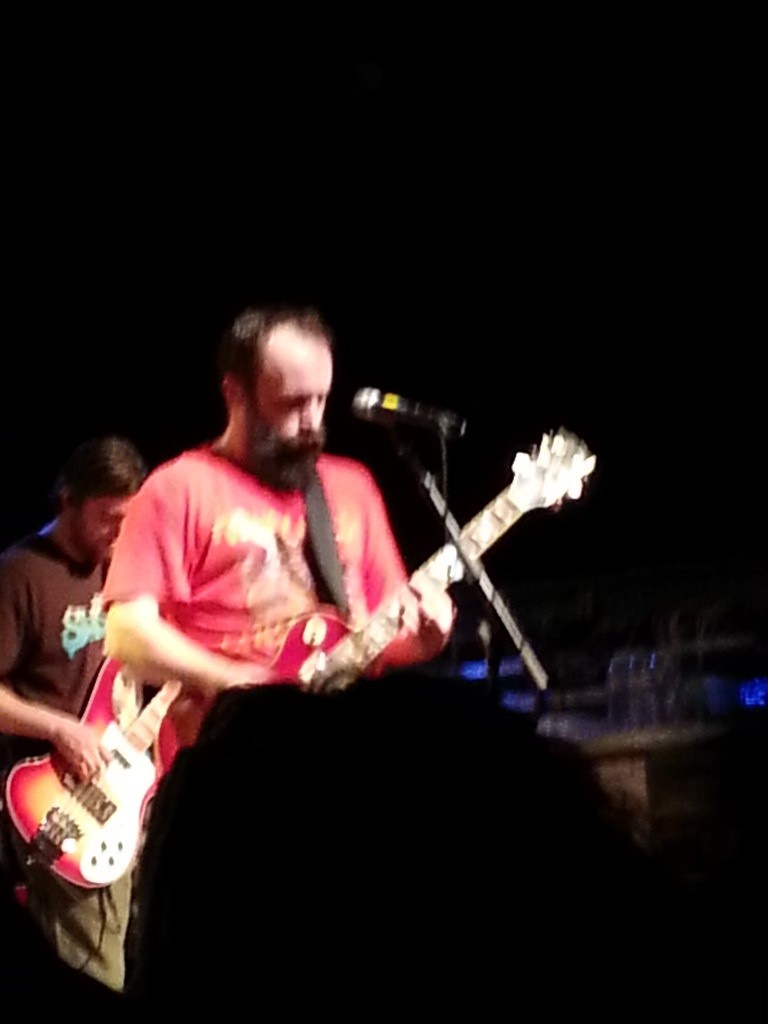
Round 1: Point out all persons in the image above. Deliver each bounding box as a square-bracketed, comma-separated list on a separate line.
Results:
[128, 656, 709, 1017]
[3, 440, 151, 994]
[103, 294, 459, 764]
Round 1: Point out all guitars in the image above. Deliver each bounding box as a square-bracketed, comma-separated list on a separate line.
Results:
[4, 647, 188, 907]
[258, 428, 603, 692]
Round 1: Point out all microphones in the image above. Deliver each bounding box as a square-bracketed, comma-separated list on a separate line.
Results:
[353, 387, 468, 439]
